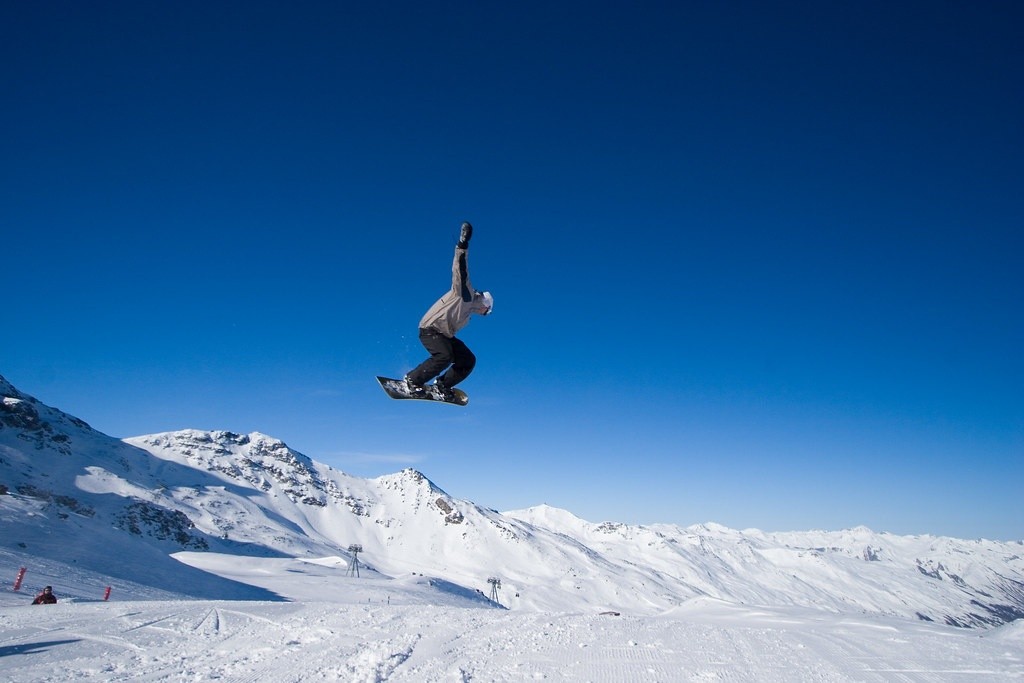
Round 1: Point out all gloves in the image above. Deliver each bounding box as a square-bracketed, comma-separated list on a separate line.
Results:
[458, 221, 472, 249]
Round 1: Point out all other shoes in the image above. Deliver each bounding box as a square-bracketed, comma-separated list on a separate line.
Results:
[403, 375, 426, 399]
[433, 378, 453, 401]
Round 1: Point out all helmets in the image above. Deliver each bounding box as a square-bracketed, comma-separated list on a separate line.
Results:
[481, 291, 493, 315]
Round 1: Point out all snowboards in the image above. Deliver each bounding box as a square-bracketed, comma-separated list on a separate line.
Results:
[374, 375, 469, 406]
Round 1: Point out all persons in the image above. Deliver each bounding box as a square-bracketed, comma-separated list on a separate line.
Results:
[32, 586, 57, 605]
[405, 222, 493, 398]
